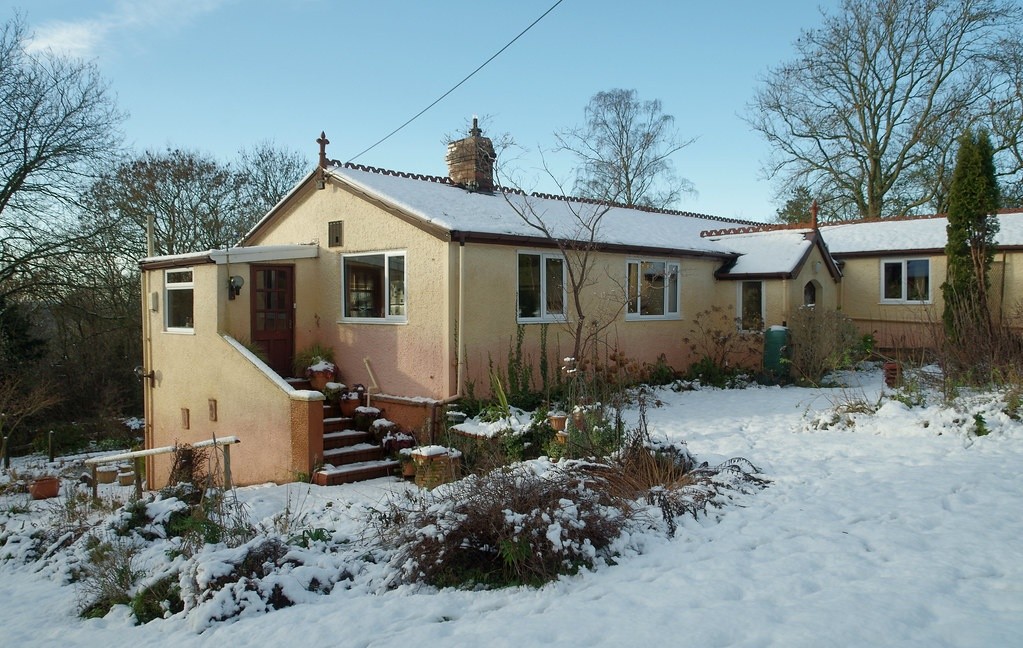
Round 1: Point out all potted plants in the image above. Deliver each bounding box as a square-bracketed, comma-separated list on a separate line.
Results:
[301, 347, 606, 488]
